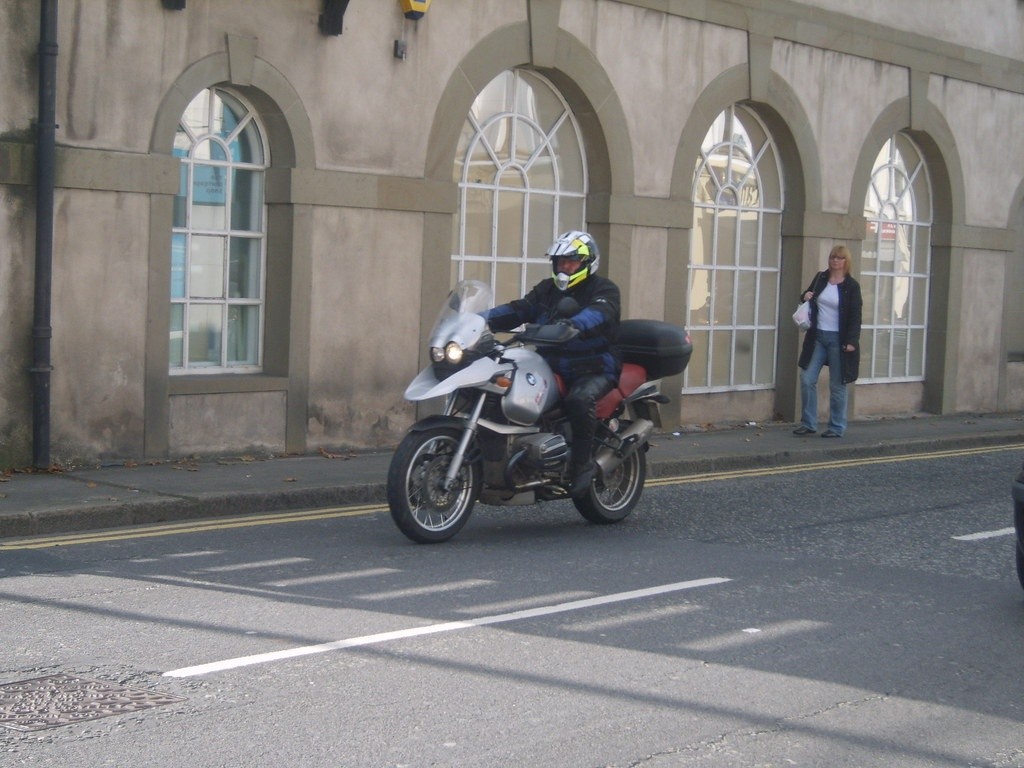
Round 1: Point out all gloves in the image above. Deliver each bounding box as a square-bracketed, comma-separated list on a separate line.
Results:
[555, 319, 574, 330]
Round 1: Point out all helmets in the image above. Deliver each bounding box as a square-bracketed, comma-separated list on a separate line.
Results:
[545, 231, 601, 291]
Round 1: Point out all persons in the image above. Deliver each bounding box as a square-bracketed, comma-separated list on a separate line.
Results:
[476, 231, 621, 497]
[793, 245, 863, 438]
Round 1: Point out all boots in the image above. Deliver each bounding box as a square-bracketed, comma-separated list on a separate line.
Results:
[569, 438, 595, 498]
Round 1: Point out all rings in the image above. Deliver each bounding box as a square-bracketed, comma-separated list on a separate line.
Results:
[809, 297, 810, 299]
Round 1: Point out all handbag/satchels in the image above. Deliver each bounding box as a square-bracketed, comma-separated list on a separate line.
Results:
[792, 302, 812, 331]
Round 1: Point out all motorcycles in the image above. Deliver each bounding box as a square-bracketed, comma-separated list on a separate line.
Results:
[387, 279, 694, 543]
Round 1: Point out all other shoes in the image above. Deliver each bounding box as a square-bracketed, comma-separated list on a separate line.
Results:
[793, 425, 816, 437]
[821, 430, 838, 437]
